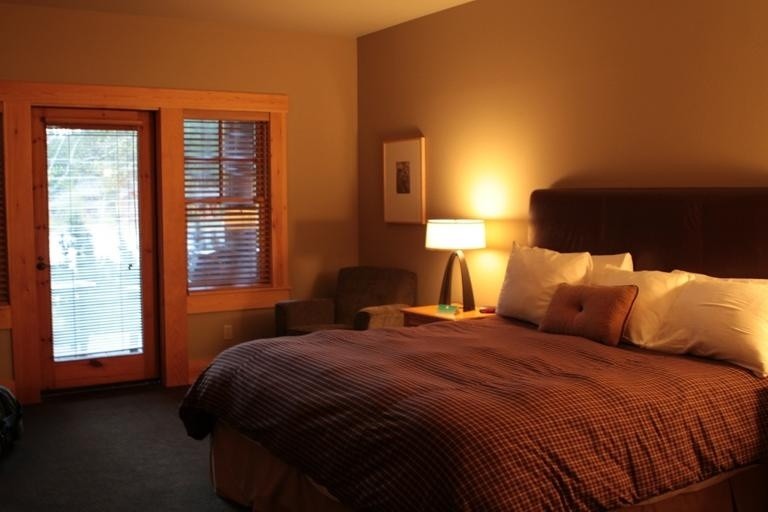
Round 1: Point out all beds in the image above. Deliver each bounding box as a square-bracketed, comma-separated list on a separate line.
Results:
[198, 185, 768, 512]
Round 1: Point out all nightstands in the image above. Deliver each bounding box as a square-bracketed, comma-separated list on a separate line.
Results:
[399, 301, 494, 328]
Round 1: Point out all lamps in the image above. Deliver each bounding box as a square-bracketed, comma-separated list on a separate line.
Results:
[422, 218, 487, 316]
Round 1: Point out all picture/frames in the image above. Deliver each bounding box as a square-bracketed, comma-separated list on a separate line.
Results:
[381, 134, 426, 227]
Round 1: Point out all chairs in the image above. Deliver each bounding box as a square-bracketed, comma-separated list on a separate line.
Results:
[266, 265, 419, 340]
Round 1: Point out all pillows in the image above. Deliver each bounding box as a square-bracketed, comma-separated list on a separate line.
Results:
[495, 238, 768, 380]
